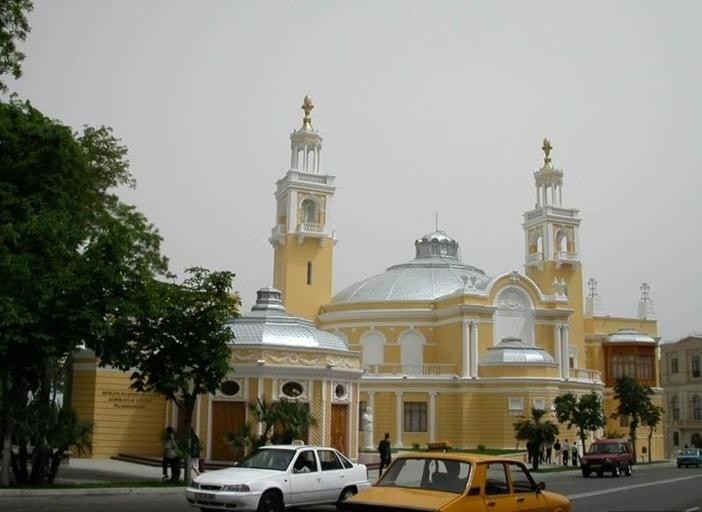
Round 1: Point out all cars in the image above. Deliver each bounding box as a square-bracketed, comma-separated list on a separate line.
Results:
[676, 446, 702, 468]
[338, 443, 572, 511]
[185, 439, 370, 509]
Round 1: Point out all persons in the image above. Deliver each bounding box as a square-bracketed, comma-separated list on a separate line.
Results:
[361, 406, 376, 449]
[189, 426, 203, 477]
[161, 425, 177, 479]
[377, 432, 392, 478]
[526, 437, 579, 466]
[294, 454, 311, 473]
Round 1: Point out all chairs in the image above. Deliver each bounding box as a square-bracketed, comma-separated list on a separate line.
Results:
[428, 467, 505, 497]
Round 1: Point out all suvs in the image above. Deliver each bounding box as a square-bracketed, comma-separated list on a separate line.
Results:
[580, 437, 636, 477]
